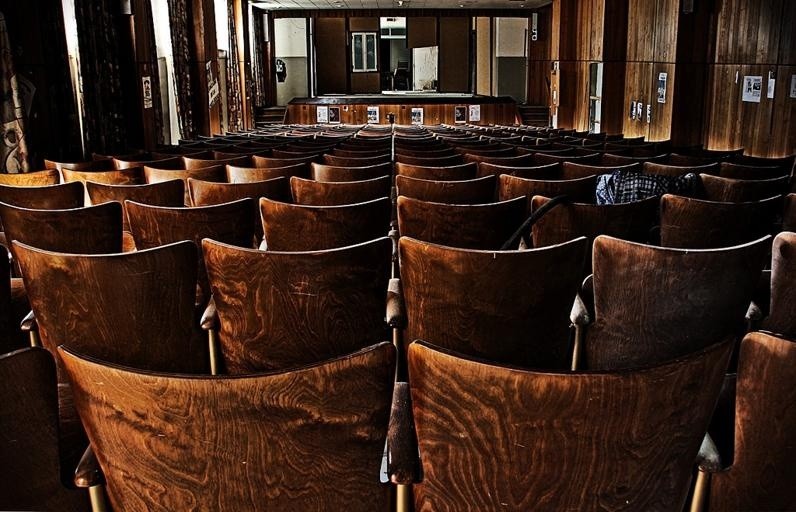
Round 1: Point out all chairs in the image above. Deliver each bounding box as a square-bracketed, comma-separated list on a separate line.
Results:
[382, 68, 410, 91]
